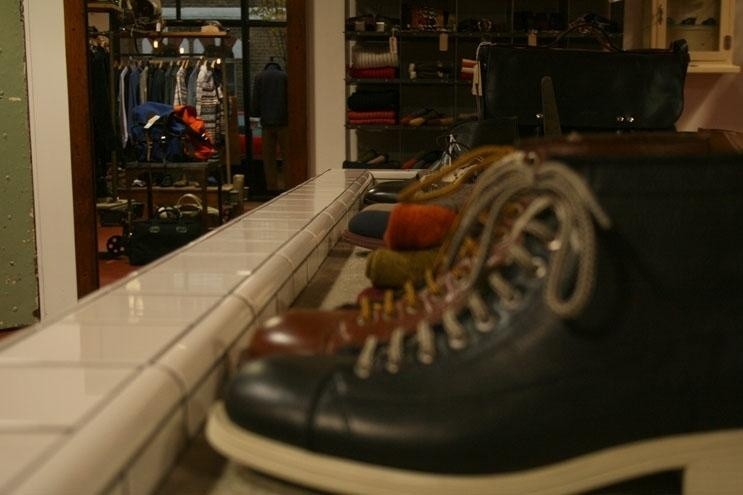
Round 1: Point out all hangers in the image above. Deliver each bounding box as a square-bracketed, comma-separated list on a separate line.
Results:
[110, 52, 220, 87]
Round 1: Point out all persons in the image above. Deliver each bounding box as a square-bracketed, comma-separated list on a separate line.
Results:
[253, 62, 290, 172]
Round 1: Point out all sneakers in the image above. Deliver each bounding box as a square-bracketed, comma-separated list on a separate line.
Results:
[131, 168, 188, 187]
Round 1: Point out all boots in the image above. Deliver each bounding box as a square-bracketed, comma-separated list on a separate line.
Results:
[249, 133, 741, 388]
[204, 148, 743, 495]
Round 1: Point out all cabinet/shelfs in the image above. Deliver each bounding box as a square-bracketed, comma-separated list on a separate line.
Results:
[343, 1, 613, 169]
[641, 0, 741, 75]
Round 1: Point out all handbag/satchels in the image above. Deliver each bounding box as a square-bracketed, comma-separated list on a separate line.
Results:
[472, 39, 690, 130]
[125, 193, 225, 265]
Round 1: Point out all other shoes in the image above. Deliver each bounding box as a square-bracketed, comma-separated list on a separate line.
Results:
[340, 169, 483, 307]
